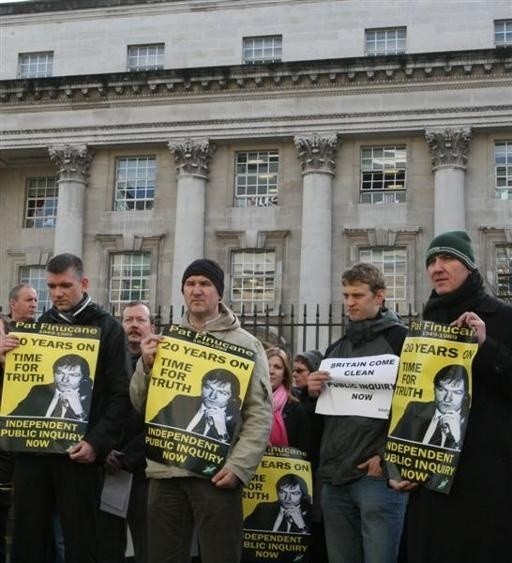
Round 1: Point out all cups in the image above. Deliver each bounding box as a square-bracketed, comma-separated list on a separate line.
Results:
[292, 369, 308, 373]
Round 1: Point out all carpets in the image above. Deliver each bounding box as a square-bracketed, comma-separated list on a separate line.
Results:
[50, 394, 62, 417]
[192, 410, 206, 434]
[429, 416, 442, 445]
[277, 511, 287, 532]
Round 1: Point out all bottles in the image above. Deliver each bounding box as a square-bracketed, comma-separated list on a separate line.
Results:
[297, 350, 323, 372]
[181, 258, 224, 296]
[426, 232, 475, 272]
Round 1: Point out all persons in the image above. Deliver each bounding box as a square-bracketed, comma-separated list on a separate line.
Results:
[148, 368, 242, 446]
[305, 261, 411, 563]
[126, 259, 274, 563]
[5, 354, 94, 423]
[389, 364, 468, 453]
[98, 301, 147, 563]
[262, 345, 308, 448]
[378, 232, 512, 563]
[6, 283, 39, 323]
[0, 314, 12, 335]
[1, 253, 124, 563]
[244, 474, 312, 533]
[289, 349, 324, 394]
